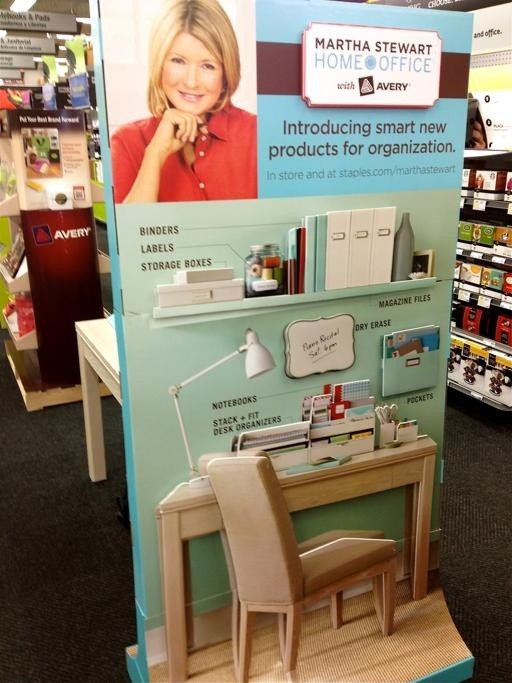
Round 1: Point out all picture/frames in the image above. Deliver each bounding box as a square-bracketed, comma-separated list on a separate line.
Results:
[412, 248, 436, 277]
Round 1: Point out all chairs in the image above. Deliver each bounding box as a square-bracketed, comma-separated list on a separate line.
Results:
[209, 457, 401, 682]
[199, 447, 386, 661]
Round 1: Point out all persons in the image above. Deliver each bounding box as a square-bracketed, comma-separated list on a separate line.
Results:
[110, 0, 258, 204]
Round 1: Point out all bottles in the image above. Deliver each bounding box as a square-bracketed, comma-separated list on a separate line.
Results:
[243, 242, 285, 296]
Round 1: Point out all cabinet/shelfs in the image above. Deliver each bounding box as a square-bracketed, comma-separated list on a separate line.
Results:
[1, 190, 39, 351]
[91, 178, 113, 317]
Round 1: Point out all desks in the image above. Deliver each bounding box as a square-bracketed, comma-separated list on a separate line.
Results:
[74, 317, 124, 482]
[154, 434, 438, 683]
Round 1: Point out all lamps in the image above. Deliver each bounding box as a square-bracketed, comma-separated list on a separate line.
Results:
[168, 325, 276, 481]
